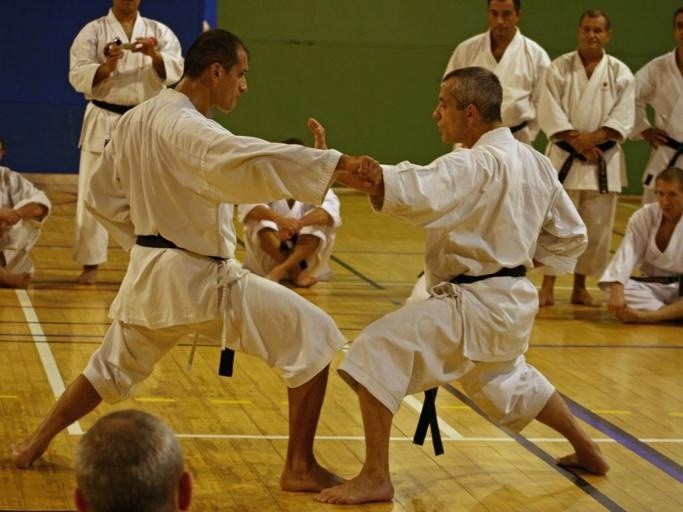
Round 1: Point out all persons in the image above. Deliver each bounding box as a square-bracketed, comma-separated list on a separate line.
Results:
[443, 0, 551, 154]
[13, 28, 384, 493]
[598, 167, 682, 324]
[635, 8, 682, 204]
[74, 410, 193, 511]
[0, 140, 52, 289]
[535, 11, 636, 307]
[68, 1, 185, 284]
[307, 67, 609, 505]
[236, 138, 343, 287]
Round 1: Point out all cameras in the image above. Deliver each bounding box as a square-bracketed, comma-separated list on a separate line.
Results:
[113, 37, 134, 51]
